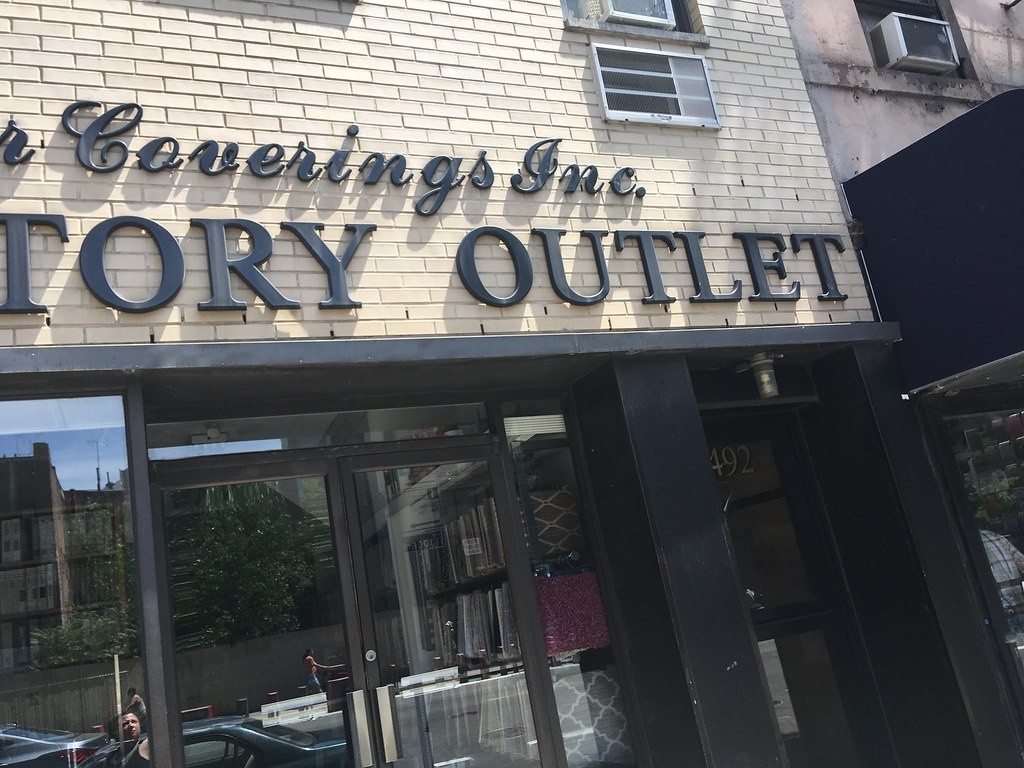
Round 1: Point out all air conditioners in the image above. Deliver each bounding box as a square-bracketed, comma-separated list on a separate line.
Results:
[867, 12, 960, 76]
[578, 0, 676, 31]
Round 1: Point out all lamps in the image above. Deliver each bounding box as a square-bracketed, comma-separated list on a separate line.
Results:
[736, 352, 783, 399]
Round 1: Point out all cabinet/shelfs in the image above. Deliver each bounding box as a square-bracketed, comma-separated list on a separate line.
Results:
[373, 421, 644, 768]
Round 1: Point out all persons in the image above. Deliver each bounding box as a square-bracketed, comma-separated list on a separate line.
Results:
[444, 621, 456, 666]
[103, 688, 150, 768]
[304, 648, 327, 696]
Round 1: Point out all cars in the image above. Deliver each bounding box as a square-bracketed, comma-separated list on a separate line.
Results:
[0, 722, 110, 768]
[77, 716, 353, 768]
